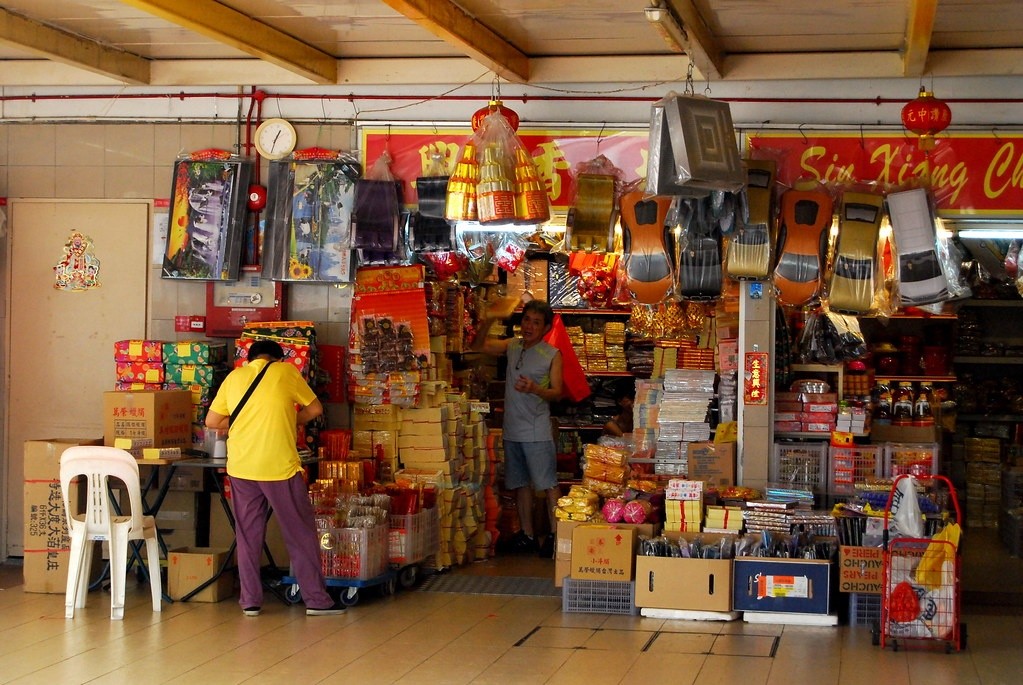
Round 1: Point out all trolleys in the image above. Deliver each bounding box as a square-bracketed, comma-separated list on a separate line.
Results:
[871, 473, 971, 657]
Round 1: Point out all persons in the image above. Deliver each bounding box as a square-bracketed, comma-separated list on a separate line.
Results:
[204, 340, 348, 615]
[470, 299, 563, 555]
[606, 380, 635, 436]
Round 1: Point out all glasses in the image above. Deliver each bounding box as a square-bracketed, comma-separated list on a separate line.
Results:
[516, 349, 525, 370]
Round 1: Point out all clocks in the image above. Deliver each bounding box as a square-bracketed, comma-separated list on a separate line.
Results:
[254, 119, 296, 160]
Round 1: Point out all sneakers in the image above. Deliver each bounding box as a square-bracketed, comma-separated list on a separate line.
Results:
[305, 602, 349, 616]
[539, 536, 554, 558]
[243, 606, 261, 616]
[511, 534, 540, 553]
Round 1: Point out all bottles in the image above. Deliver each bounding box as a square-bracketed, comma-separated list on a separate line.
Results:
[875, 380, 936, 427]
[838, 395, 873, 433]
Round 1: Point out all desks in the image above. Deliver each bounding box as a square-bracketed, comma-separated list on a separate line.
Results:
[169, 455, 326, 601]
[86, 456, 193, 604]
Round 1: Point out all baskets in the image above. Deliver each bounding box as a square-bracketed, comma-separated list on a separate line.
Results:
[770, 441, 827, 494]
[884, 442, 940, 490]
[315, 522, 390, 581]
[387, 503, 441, 565]
[562, 577, 639, 616]
[829, 445, 883, 482]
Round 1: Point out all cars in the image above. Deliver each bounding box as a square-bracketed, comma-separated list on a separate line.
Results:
[825, 189, 886, 318]
[618, 191, 675, 305]
[676, 199, 724, 300]
[881, 188, 952, 307]
[771, 179, 834, 308]
[726, 160, 778, 283]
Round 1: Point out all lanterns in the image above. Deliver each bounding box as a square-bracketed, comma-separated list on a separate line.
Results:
[472, 101, 519, 143]
[901, 92, 952, 150]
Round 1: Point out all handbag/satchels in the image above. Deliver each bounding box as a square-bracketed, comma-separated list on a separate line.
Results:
[886, 523, 958, 639]
[890, 474, 925, 538]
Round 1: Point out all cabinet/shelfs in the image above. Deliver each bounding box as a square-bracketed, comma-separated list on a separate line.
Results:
[951, 298, 1023, 424]
[482, 304, 661, 486]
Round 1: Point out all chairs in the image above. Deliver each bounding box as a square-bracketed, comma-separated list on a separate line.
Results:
[60, 447, 165, 623]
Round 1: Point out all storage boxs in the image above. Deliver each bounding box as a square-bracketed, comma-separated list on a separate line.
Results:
[20, 156, 503, 598]
[554, 326, 958, 626]
[505, 262, 548, 306]
